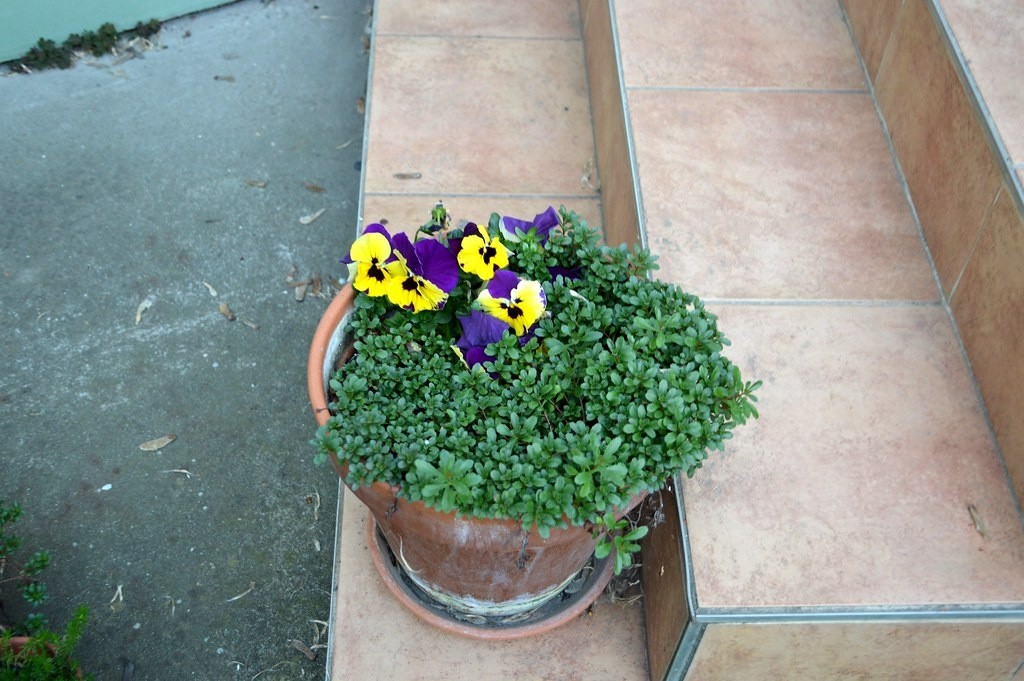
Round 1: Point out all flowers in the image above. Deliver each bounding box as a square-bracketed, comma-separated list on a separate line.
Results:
[309, 197, 766, 575]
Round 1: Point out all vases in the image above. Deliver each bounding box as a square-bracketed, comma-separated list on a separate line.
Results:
[310, 278, 652, 642]
